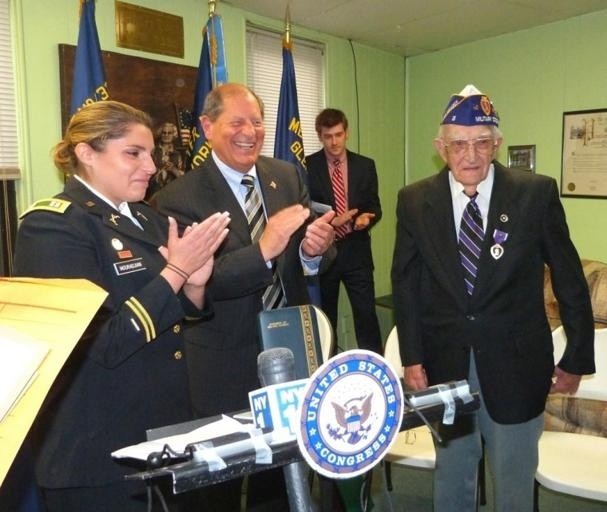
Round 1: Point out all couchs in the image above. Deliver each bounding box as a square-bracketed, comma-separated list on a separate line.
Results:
[543, 260, 607, 440]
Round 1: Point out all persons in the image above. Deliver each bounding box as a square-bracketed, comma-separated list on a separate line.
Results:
[17, 101, 232, 512]
[391, 84, 594, 512]
[303, 108, 382, 359]
[151, 121, 183, 194]
[148, 84, 338, 512]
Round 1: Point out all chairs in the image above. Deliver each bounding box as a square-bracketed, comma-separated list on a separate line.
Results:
[533, 325, 607, 512]
[383, 324, 487, 507]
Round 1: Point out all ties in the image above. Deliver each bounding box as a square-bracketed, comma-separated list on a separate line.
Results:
[330, 160, 348, 242]
[240, 174, 288, 312]
[458, 192, 484, 295]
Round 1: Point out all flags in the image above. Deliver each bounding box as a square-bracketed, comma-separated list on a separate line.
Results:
[70, 0, 111, 115]
[179, 108, 192, 146]
[188, 14, 229, 167]
[271, 48, 310, 196]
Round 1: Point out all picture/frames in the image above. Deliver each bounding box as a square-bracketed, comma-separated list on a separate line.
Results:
[559, 108, 606, 199]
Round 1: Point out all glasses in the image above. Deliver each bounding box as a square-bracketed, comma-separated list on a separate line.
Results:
[441, 137, 497, 158]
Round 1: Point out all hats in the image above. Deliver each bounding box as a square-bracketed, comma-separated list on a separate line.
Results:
[438, 84, 500, 126]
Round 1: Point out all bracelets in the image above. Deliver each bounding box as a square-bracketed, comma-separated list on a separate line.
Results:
[166, 263, 189, 280]
